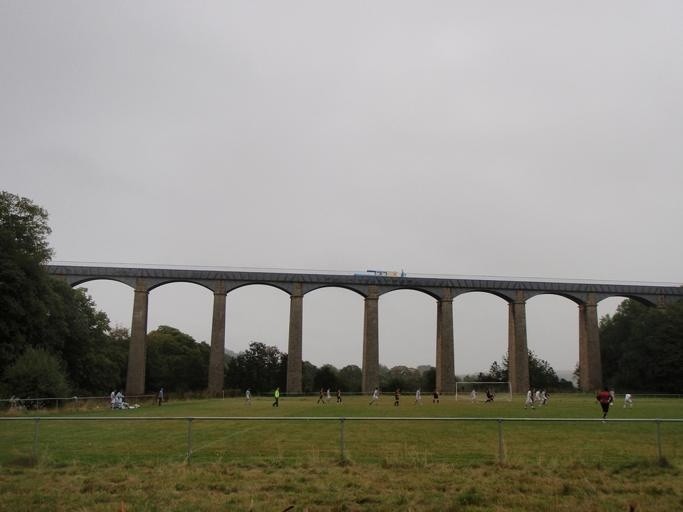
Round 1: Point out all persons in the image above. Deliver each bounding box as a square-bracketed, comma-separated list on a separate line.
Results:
[415, 386, 423, 406]
[393, 388, 401, 407]
[244, 388, 252, 405]
[9, 394, 33, 410]
[471, 387, 496, 404]
[368, 387, 379, 405]
[325, 388, 332, 405]
[432, 389, 439, 403]
[524, 386, 550, 409]
[72, 393, 78, 402]
[110, 389, 140, 410]
[594, 387, 632, 425]
[158, 388, 164, 406]
[336, 389, 343, 405]
[315, 387, 325, 406]
[272, 387, 280, 407]
